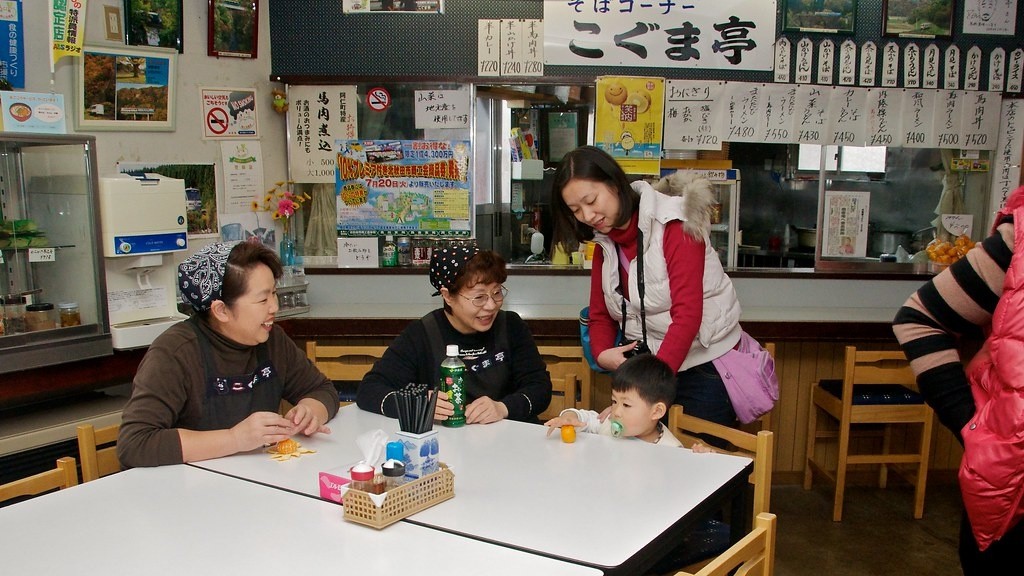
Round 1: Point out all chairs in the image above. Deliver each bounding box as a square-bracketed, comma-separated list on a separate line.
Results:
[804, 344, 934, 522]
[666, 343, 776, 576]
[306, 342, 390, 408]
[76, 423, 120, 482]
[537, 344, 595, 417]
[0, 457, 78, 507]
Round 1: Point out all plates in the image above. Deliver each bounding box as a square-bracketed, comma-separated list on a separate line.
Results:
[665, 142, 730, 159]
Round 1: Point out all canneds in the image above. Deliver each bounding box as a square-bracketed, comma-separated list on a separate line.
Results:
[0, 297, 82, 337]
[710, 203, 721, 224]
[397, 237, 411, 265]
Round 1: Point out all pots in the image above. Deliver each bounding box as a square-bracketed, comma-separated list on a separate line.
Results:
[791, 224, 817, 247]
[872, 231, 913, 254]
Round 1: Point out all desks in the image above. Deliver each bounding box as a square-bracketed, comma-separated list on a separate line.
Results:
[0, 464, 606, 576]
[185, 400, 756, 576]
[737, 248, 816, 267]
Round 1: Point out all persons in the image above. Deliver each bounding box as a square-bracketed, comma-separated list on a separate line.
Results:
[355, 240, 553, 426]
[544, 354, 718, 453]
[541, 144, 743, 456]
[891, 185, 1024, 576]
[116, 238, 340, 473]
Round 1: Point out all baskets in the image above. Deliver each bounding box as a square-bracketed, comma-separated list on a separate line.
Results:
[342, 462, 455, 530]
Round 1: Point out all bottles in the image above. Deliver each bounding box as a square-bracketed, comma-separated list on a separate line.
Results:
[0, 296, 27, 333]
[440, 344, 467, 428]
[25, 302, 56, 332]
[387, 442, 406, 480]
[411, 236, 470, 260]
[58, 301, 81, 328]
[382, 462, 406, 494]
[350, 464, 375, 495]
[383, 236, 397, 267]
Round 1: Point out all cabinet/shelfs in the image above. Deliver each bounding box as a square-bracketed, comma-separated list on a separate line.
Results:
[0, 130, 114, 373]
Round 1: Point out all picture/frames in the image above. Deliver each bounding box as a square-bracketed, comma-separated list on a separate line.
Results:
[102, 4, 123, 41]
[207, 0, 259, 58]
[123, 0, 184, 54]
[73, 42, 179, 132]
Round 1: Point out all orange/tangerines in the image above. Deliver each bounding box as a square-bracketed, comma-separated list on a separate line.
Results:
[561, 425, 576, 443]
[926, 234, 976, 264]
[277, 440, 297, 454]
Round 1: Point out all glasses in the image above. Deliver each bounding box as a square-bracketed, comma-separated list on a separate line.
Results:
[457, 285, 510, 308]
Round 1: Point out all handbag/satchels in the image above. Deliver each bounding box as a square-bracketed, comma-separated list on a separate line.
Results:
[711, 330, 780, 423]
[580, 306, 620, 375]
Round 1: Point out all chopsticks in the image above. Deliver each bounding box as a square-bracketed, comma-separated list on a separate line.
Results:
[393, 383, 439, 434]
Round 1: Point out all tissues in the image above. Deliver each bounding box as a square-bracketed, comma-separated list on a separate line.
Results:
[319, 428, 392, 505]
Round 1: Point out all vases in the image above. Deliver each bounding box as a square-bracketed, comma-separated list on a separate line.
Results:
[280, 233, 295, 267]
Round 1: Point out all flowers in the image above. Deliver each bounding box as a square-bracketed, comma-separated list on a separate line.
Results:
[250, 180, 312, 250]
